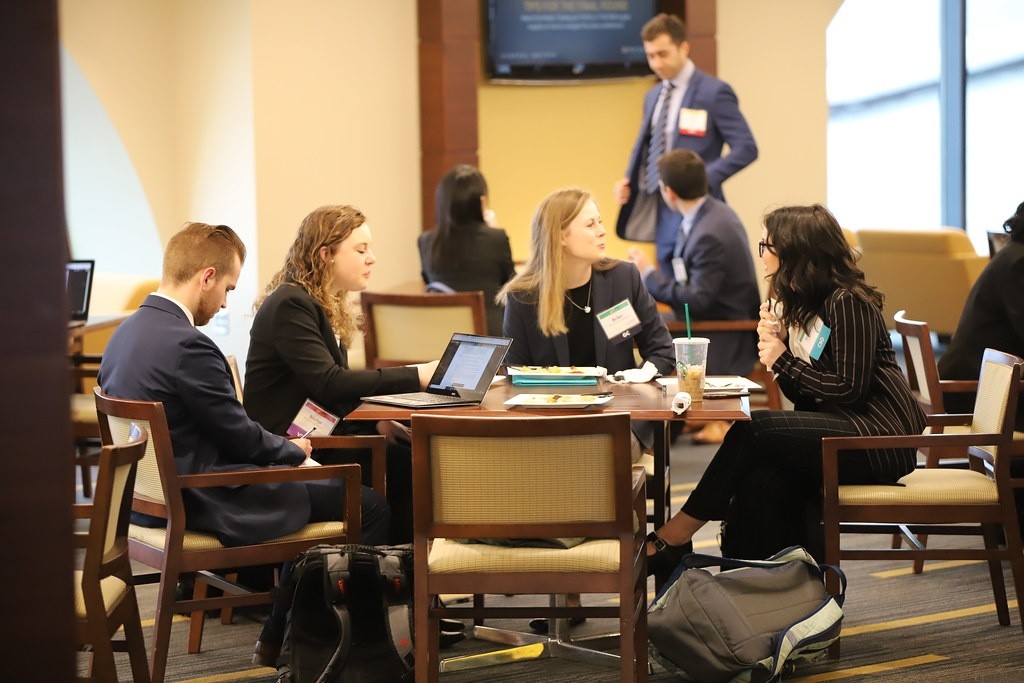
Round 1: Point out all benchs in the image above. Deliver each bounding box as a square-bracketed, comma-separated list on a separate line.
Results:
[854, 228, 990, 344]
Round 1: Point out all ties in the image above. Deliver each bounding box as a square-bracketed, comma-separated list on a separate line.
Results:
[673, 222, 684, 259]
[644, 82, 675, 195]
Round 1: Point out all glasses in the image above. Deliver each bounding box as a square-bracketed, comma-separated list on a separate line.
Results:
[758, 241, 776, 258]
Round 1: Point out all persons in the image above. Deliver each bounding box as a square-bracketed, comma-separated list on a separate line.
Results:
[243, 205, 467, 649]
[495, 190, 675, 630]
[417, 166, 518, 336]
[645, 203, 928, 579]
[96, 223, 391, 667]
[937, 202, 1024, 544]
[614, 13, 757, 443]
[628, 149, 762, 374]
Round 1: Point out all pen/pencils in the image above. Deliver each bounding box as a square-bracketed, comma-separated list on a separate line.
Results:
[301, 427, 318, 439]
[580, 391, 613, 396]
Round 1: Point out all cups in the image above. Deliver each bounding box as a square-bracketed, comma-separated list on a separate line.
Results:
[672, 337, 710, 402]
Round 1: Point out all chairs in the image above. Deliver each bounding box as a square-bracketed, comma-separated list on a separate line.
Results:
[806, 347, 1024, 661]
[666, 284, 783, 410]
[73, 422, 151, 682]
[68, 355, 103, 496]
[638, 420, 671, 598]
[92, 385, 361, 683]
[410, 414, 649, 683]
[894, 310, 1024, 575]
[361, 291, 487, 371]
[220, 355, 387, 623]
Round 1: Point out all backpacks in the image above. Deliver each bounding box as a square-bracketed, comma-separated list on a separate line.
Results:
[276, 544, 414, 683]
[643, 548, 847, 683]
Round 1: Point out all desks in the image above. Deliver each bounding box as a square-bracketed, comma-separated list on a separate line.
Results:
[343, 374, 751, 674]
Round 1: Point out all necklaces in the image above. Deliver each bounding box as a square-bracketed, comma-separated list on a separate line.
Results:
[564, 280, 591, 313]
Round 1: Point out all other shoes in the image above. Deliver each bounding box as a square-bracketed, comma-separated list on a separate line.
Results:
[644, 530, 693, 576]
[434, 629, 466, 649]
[251, 639, 279, 668]
[530, 604, 587, 632]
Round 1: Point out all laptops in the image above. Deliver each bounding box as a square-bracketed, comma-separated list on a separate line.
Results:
[359, 333, 513, 409]
[65, 259, 95, 328]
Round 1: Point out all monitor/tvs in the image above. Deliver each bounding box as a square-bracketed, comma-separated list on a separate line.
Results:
[478, 0, 685, 85]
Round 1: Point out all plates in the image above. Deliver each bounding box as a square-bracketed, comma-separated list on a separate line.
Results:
[507, 366, 601, 379]
[656, 378, 763, 391]
